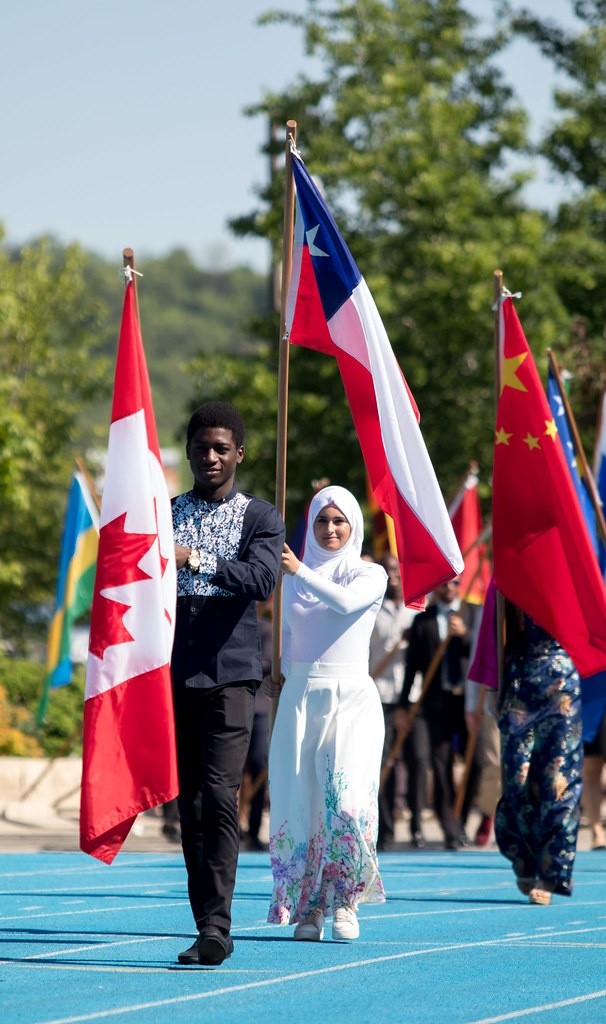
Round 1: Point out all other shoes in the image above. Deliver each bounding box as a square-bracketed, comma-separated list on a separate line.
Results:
[447, 837, 460, 850]
[249, 840, 266, 852]
[476, 819, 490, 845]
[412, 831, 425, 849]
[163, 826, 181, 844]
[530, 886, 551, 905]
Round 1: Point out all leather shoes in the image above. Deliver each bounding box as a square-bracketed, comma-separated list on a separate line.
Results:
[177, 926, 233, 963]
[197, 926, 227, 965]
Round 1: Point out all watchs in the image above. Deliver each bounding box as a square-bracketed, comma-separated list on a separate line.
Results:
[185, 549, 200, 571]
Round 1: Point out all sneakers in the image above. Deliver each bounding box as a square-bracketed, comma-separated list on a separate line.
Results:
[332, 903, 360, 940]
[294, 907, 326, 942]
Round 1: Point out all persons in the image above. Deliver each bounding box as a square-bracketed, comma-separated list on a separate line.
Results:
[170, 400, 286, 965]
[495, 589, 582, 905]
[267, 486, 387, 941]
[162, 551, 606, 850]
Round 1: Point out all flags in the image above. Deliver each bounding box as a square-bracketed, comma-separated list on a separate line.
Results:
[291, 472, 491, 605]
[36, 478, 101, 726]
[547, 369, 606, 555]
[285, 152, 464, 612]
[491, 291, 606, 679]
[78, 280, 181, 865]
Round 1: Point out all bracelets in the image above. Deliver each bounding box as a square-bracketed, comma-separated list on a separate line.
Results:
[486, 689, 498, 692]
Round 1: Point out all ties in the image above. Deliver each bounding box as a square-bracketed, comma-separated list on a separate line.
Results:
[445, 609, 463, 687]
